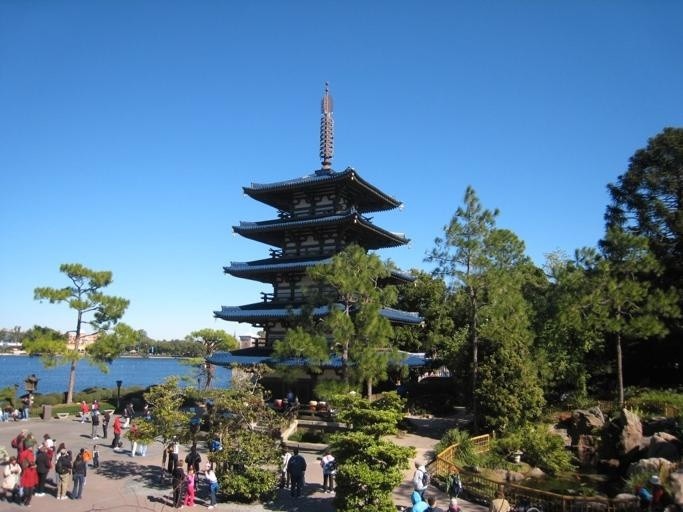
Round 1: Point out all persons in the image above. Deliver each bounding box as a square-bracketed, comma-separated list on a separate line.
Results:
[279, 446, 306, 498]
[488, 490, 510, 511]
[0, 430, 92, 506]
[633, 482, 651, 512]
[320, 448, 335, 493]
[166, 435, 218, 511]
[411, 461, 462, 512]
[648, 475, 667, 511]
[81, 400, 153, 457]
[6, 396, 29, 422]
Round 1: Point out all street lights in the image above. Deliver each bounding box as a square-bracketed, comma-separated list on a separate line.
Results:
[116, 380, 122, 410]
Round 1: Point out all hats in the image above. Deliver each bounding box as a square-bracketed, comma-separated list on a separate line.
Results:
[648, 475, 660, 484]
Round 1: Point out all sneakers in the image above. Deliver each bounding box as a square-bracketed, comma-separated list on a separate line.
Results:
[34, 492, 46, 497]
[57, 496, 81, 500]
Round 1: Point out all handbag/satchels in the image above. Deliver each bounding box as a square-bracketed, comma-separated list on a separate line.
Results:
[326, 461, 338, 472]
[2, 476, 15, 490]
[55, 462, 63, 474]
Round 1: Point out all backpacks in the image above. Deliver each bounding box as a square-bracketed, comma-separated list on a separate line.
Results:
[451, 474, 463, 496]
[418, 469, 432, 487]
